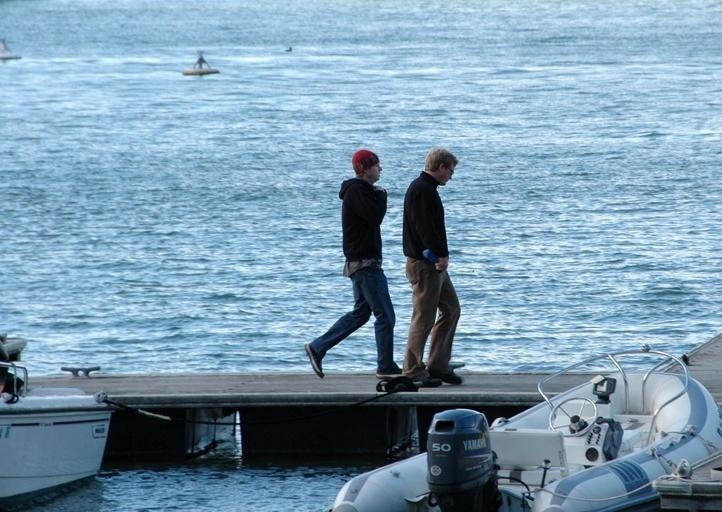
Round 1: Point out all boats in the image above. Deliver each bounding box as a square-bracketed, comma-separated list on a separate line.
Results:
[179, 65, 219, 77]
[323, 345, 722, 512]
[0, 54, 24, 61]
[0, 329, 119, 510]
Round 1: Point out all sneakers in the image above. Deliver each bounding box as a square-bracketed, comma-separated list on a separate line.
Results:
[376, 368, 463, 387]
[305, 344, 325, 379]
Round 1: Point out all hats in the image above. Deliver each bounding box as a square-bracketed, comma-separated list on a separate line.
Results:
[352, 150, 380, 176]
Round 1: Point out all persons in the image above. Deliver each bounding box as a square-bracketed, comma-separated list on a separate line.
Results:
[401, 146, 467, 385]
[305, 150, 401, 378]
